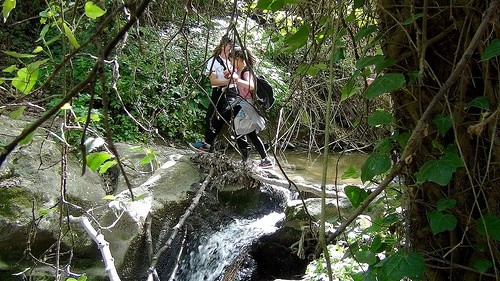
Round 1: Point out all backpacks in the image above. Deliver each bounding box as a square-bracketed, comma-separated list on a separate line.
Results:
[241, 66, 276, 111]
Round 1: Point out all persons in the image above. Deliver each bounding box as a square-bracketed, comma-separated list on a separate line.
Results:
[189, 46, 273, 168]
[203, 35, 251, 161]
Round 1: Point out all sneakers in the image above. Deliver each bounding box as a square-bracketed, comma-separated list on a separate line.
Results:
[189, 142, 211, 153]
[257, 158, 273, 168]
